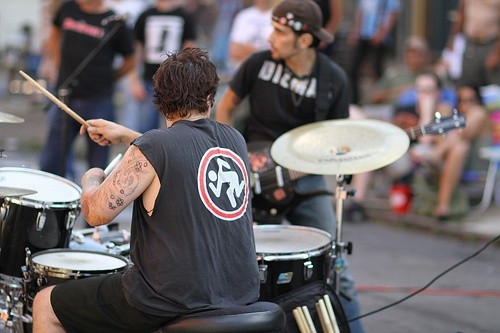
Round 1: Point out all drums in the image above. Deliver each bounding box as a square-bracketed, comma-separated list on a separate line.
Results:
[0, 167, 83, 283]
[254, 224, 337, 302]
[23, 247, 130, 317]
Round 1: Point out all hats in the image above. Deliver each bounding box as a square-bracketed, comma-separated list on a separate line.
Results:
[271, 0, 335, 43]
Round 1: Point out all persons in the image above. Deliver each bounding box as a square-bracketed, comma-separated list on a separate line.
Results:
[215, 1, 365, 333]
[32, 48, 261, 333]
[229, 0, 282, 61]
[346, 0, 402, 82]
[385, 0, 500, 224]
[39, 0, 145, 230]
[135, 0, 198, 135]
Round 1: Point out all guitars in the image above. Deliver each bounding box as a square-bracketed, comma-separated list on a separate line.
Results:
[247, 107, 467, 220]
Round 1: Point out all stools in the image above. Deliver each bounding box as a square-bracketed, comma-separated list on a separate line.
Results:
[151, 302, 286, 333]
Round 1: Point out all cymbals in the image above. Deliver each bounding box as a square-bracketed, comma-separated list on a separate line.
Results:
[269, 119, 411, 176]
[0, 187, 38, 198]
[0, 112, 25, 123]
